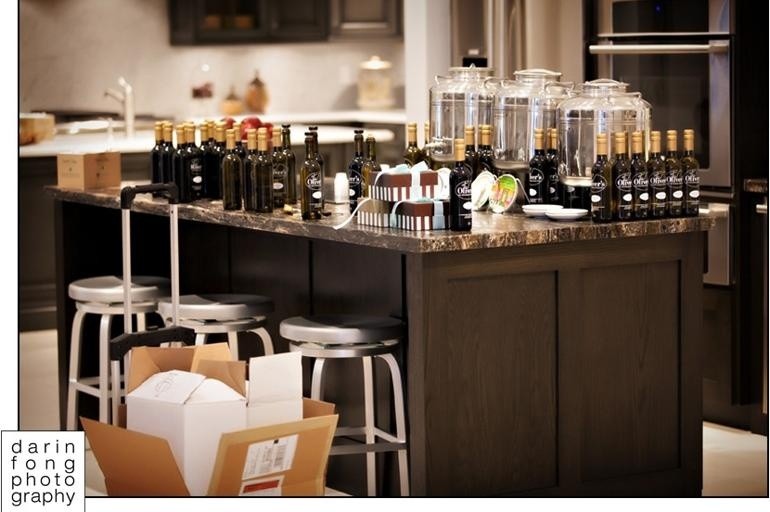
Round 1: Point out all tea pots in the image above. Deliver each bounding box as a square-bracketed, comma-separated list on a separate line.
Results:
[356, 54, 396, 111]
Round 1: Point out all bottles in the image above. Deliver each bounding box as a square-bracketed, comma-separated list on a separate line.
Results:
[403, 121, 422, 166]
[299, 125, 327, 222]
[526, 128, 563, 203]
[192, 64, 268, 117]
[418, 118, 429, 163]
[591, 127, 701, 224]
[151, 118, 296, 214]
[449, 123, 497, 231]
[349, 128, 382, 216]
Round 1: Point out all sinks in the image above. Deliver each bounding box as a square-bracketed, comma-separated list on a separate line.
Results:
[54, 119, 176, 136]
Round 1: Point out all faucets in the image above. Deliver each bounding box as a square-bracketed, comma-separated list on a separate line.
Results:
[104, 75, 138, 120]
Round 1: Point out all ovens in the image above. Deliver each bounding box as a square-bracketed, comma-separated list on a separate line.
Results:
[594, 0, 737, 288]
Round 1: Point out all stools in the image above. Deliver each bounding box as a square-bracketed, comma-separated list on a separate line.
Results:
[66, 274, 410, 498]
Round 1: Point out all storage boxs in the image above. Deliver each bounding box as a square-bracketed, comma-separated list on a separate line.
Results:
[80, 342, 339, 497]
[19, 113, 56, 145]
[57, 150, 122, 192]
[357, 168, 451, 230]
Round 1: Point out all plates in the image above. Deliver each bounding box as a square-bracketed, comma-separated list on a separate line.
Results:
[521, 204, 589, 220]
